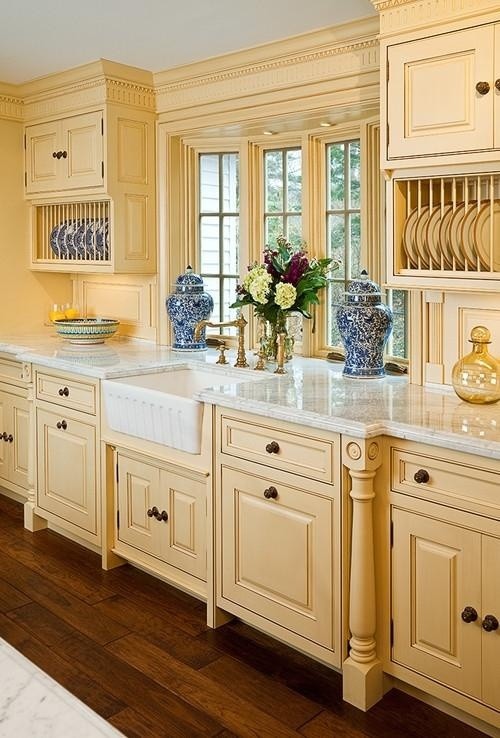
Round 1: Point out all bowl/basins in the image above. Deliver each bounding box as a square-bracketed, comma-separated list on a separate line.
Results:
[56, 318, 120, 345]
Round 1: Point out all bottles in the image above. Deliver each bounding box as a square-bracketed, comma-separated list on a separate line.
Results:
[453, 327, 498, 402]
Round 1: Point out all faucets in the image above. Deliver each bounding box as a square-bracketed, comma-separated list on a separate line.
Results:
[193, 313, 250, 368]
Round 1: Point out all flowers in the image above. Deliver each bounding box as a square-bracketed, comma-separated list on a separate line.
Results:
[229, 234, 342, 333]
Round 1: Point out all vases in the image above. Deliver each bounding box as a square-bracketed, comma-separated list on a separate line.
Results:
[262, 312, 302, 363]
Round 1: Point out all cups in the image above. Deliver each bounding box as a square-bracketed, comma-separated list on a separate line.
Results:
[66, 305, 81, 316]
[49, 304, 66, 337]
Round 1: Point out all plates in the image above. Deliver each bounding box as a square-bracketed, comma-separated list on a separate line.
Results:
[404, 203, 428, 269]
[415, 203, 440, 270]
[50, 220, 66, 259]
[426, 204, 453, 270]
[92, 221, 109, 260]
[449, 200, 480, 271]
[439, 203, 465, 271]
[474, 201, 500, 271]
[73, 219, 89, 260]
[56, 219, 71, 260]
[82, 219, 108, 260]
[64, 218, 80, 259]
[462, 203, 495, 272]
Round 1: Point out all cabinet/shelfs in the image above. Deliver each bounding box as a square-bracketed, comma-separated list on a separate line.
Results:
[33, 362, 102, 569]
[372, 1, 500, 291]
[103, 429, 215, 629]
[213, 405, 382, 713]
[382, 436, 500, 737]
[22, 58, 156, 273]
[0, 352, 33, 532]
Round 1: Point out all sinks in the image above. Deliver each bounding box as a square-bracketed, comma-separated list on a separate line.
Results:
[100, 368, 259, 455]
